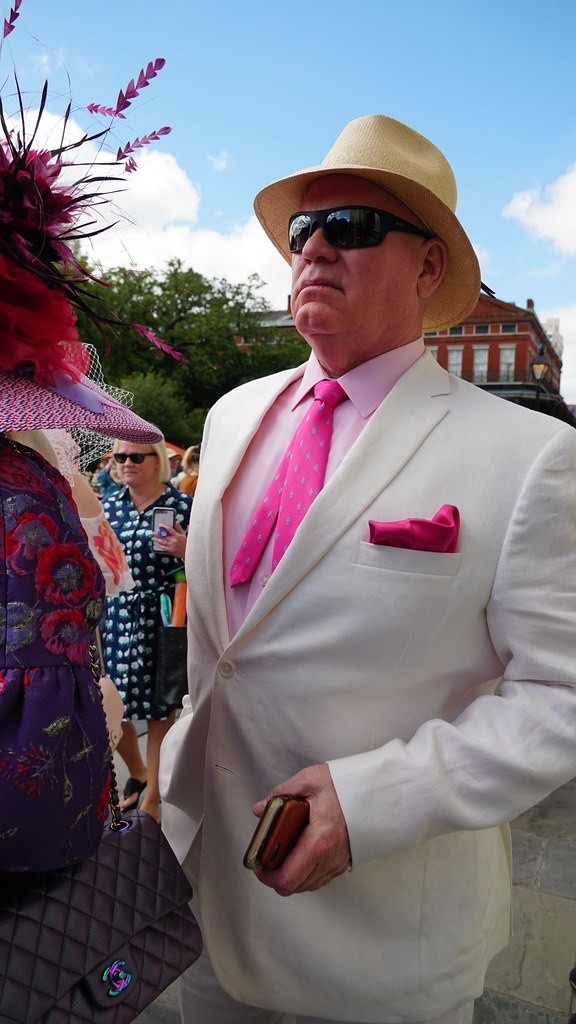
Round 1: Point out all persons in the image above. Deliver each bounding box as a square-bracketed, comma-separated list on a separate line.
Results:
[39, 425, 133, 789]
[76, 439, 200, 501]
[144, 116, 574, 1023]
[0, 277, 109, 955]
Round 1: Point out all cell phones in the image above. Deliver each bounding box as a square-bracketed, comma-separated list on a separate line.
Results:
[246, 797, 310, 879]
[150, 507, 176, 554]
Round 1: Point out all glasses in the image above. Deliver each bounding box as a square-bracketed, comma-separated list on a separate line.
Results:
[113, 451, 157, 463]
[287, 205, 433, 253]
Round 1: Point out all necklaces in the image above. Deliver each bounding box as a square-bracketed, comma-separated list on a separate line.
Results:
[129, 482, 162, 508]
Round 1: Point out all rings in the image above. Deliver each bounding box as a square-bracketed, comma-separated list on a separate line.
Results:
[164, 540, 167, 545]
[86, 417, 207, 832]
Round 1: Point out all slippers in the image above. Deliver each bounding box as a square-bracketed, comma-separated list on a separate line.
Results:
[121, 778, 148, 813]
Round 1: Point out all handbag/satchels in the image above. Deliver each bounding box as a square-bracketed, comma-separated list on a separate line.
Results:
[152, 625, 188, 721]
[0, 808, 207, 1023]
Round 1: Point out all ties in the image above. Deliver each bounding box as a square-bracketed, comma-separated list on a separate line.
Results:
[229, 379, 346, 590]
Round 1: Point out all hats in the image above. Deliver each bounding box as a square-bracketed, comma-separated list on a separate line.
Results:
[254, 114, 480, 332]
[0, 357, 163, 445]
[166, 447, 180, 459]
